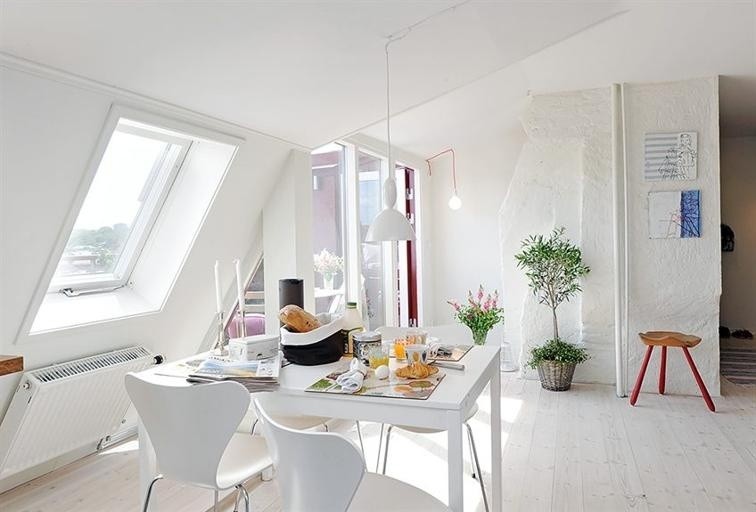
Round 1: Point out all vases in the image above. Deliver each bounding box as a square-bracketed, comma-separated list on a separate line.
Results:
[323, 273, 335, 290]
[470, 325, 490, 345]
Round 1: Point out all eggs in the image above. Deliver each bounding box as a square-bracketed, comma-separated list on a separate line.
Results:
[374, 365, 389, 379]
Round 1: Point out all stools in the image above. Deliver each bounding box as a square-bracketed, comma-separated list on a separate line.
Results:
[629, 331, 716, 412]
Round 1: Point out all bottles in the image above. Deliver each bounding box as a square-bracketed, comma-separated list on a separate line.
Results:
[351, 330, 383, 367]
[338, 301, 363, 358]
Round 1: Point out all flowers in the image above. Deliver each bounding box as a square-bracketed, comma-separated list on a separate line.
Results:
[447, 287, 504, 329]
[312, 248, 343, 274]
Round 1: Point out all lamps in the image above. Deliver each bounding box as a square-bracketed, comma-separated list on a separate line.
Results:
[364, 38, 417, 244]
[448, 150, 462, 210]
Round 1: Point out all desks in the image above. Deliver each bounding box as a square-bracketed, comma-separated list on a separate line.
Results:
[314, 288, 345, 316]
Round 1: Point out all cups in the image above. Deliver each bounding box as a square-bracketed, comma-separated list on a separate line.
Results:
[405, 327, 430, 348]
[393, 335, 414, 360]
[366, 346, 389, 369]
[403, 344, 431, 367]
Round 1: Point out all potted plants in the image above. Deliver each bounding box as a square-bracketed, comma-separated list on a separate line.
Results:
[514, 227, 592, 393]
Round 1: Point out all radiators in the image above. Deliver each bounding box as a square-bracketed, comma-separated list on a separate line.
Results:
[0, 344, 165, 481]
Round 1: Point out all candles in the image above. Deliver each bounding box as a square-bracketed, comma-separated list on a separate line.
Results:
[215, 260, 223, 310]
[234, 257, 245, 309]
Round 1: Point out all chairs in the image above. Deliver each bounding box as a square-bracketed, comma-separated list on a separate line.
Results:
[122, 373, 276, 512]
[253, 327, 368, 472]
[376, 324, 489, 512]
[239, 291, 265, 318]
[329, 272, 366, 317]
[254, 399, 457, 512]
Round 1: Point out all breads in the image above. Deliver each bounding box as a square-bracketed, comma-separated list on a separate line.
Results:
[424, 364, 438, 376]
[277, 304, 324, 333]
[395, 362, 428, 378]
